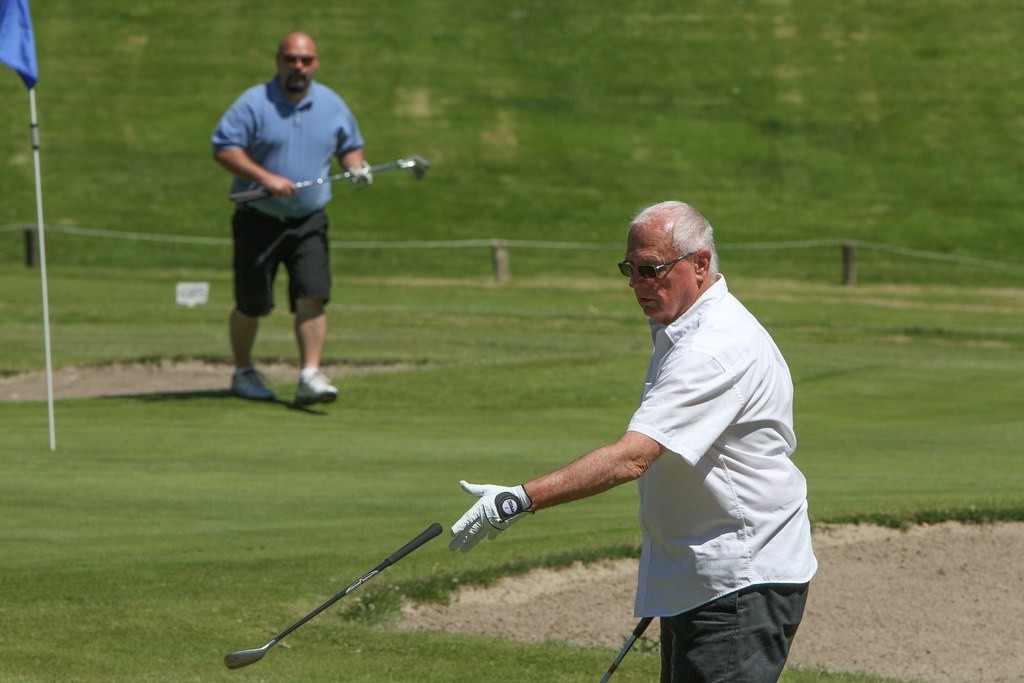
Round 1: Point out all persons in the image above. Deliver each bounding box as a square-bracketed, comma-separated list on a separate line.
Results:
[210, 32, 375, 407]
[450, 201, 818, 683]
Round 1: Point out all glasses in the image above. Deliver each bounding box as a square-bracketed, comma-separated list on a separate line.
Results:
[618, 251, 695, 278]
[281, 56, 317, 66]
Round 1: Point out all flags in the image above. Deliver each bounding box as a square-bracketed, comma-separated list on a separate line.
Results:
[0, 0, 37, 91]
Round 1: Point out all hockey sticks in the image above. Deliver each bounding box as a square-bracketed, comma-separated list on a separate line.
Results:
[229, 154, 429, 202]
[223, 521, 444, 668]
[601, 617, 653, 683]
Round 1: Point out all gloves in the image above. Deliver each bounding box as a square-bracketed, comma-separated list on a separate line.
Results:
[448, 478, 535, 554]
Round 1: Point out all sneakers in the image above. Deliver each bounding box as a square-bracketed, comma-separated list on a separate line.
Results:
[230, 367, 277, 400]
[295, 368, 338, 407]
